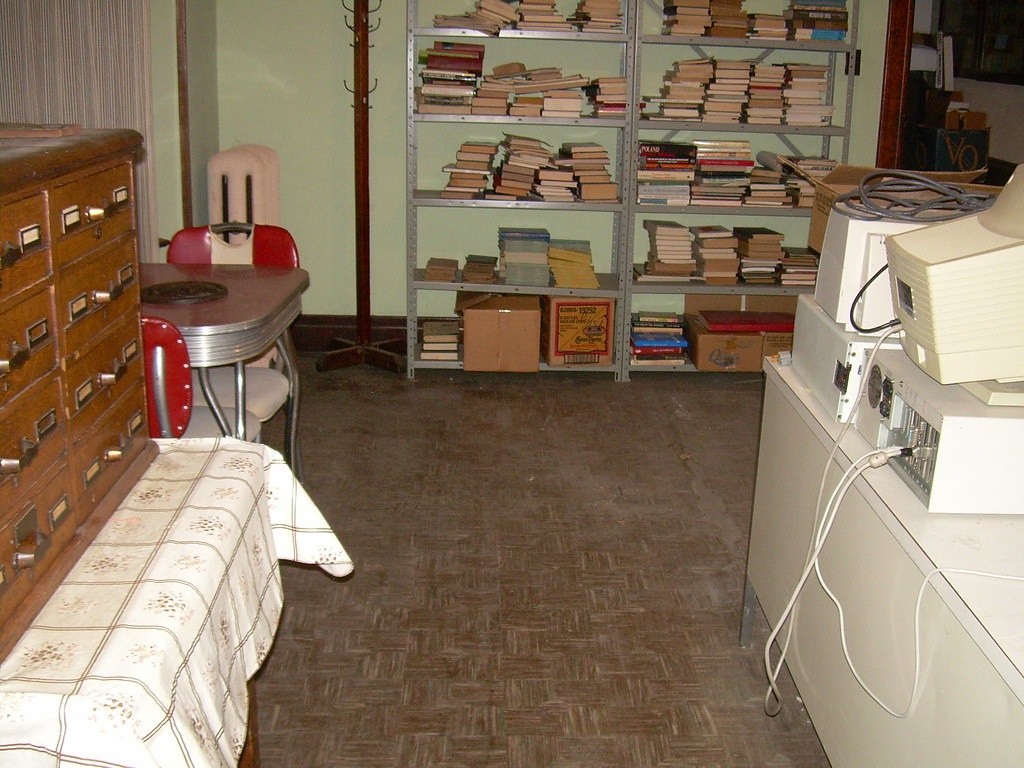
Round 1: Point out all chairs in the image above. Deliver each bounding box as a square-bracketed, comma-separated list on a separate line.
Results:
[167, 223, 305, 485]
[140, 316, 193, 439]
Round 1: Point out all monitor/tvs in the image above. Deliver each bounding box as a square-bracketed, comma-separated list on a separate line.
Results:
[883, 165, 1023, 407]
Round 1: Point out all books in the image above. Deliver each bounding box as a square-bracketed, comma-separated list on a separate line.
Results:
[498, 228, 601, 288]
[661, 0, 848, 44]
[421, 321, 460, 360]
[433, 0, 625, 33]
[697, 310, 795, 332]
[629, 311, 689, 365]
[441, 132, 619, 201]
[461, 254, 498, 283]
[633, 219, 818, 286]
[423, 257, 459, 283]
[642, 56, 835, 127]
[415, 41, 646, 118]
[638, 139, 837, 207]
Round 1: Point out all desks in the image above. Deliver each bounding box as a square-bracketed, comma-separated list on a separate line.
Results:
[139, 261, 311, 477]
[0, 437, 354, 768]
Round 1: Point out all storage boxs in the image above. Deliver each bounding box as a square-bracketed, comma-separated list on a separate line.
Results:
[908, 122, 992, 185]
[682, 293, 763, 373]
[541, 295, 616, 368]
[924, 90, 986, 130]
[453, 291, 541, 373]
[744, 295, 797, 369]
[775, 153, 1004, 254]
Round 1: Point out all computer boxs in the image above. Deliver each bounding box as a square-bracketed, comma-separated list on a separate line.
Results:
[857, 349, 1024, 516]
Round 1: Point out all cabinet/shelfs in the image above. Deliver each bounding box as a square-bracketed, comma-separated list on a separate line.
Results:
[0, 129, 161, 665]
[405, 0, 637, 382]
[737, 357, 1024, 768]
[622, 0, 860, 382]
